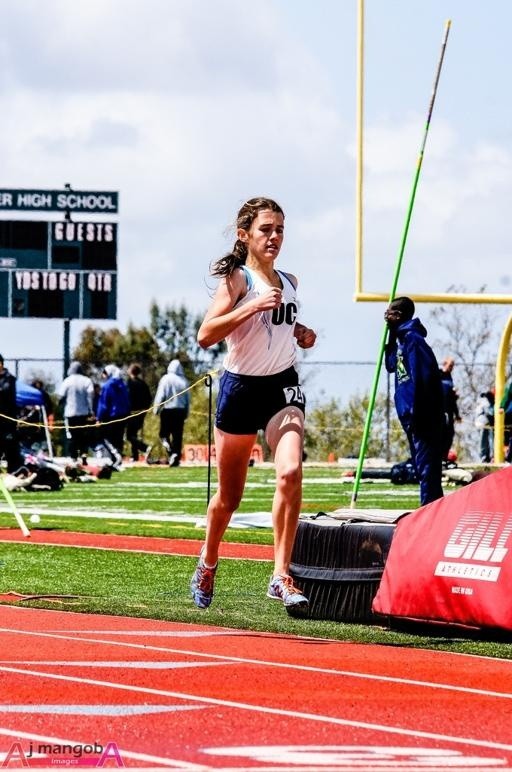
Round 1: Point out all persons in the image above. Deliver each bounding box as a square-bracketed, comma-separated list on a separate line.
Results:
[151, 358, 191, 468]
[382, 296, 448, 507]
[436, 357, 463, 461]
[0, 353, 154, 474]
[189, 195, 316, 611]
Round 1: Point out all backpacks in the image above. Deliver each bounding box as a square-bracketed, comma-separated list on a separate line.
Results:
[0, 461, 117, 492]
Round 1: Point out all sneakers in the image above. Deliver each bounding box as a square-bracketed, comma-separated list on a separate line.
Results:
[71, 438, 181, 467]
[189, 544, 218, 609]
[266, 575, 309, 608]
